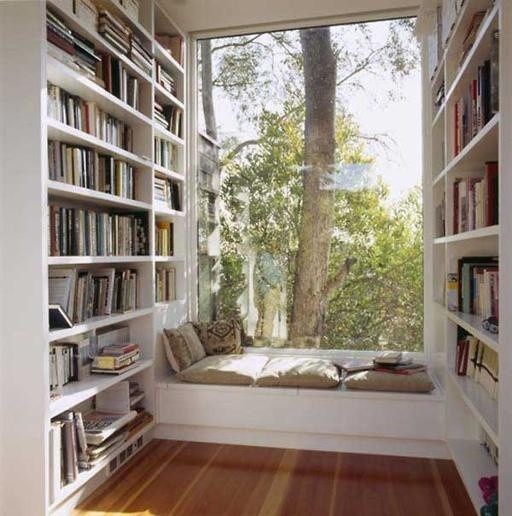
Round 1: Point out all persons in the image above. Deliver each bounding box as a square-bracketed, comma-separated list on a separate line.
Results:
[251, 238, 284, 342]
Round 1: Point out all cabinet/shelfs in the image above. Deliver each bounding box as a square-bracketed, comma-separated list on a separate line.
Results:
[411, 0, 512, 516]
[0, 0, 191, 516]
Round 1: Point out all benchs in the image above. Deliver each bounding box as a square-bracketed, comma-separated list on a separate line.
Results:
[152, 352, 453, 461]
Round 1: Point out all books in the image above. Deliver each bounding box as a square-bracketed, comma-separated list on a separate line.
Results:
[50, 206, 149, 256]
[154, 30, 185, 305]
[49, 324, 142, 398]
[426, 0, 502, 398]
[52, 379, 154, 485]
[47, 6, 141, 113]
[52, 0, 152, 78]
[329, 352, 427, 375]
[48, 267, 138, 330]
[476, 428, 498, 468]
[48, 138, 136, 201]
[47, 80, 133, 154]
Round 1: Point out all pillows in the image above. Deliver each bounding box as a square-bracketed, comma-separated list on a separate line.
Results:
[175, 355, 271, 387]
[254, 355, 341, 389]
[161, 322, 206, 373]
[189, 318, 246, 357]
[342, 363, 437, 392]
